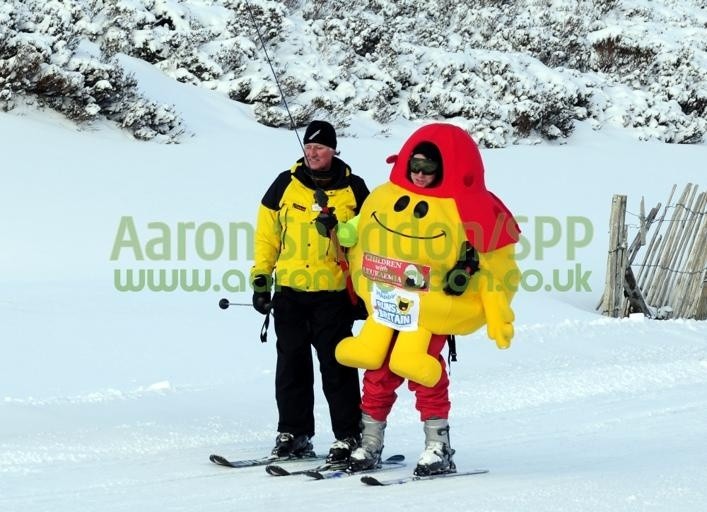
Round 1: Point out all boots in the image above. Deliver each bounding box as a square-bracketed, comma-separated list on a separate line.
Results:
[417, 416, 456, 475]
[350, 413, 387, 467]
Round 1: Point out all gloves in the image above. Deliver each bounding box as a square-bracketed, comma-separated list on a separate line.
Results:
[252, 291, 274, 314]
[315, 207, 339, 237]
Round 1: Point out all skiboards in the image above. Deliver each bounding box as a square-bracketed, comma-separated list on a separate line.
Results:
[206, 453, 360, 478]
[304, 452, 491, 484]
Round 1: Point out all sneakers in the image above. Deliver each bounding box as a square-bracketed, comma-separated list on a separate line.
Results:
[271, 433, 313, 457]
[326, 434, 362, 464]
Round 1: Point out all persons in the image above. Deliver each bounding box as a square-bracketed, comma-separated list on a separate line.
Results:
[245, 119, 368, 464]
[314, 140, 514, 478]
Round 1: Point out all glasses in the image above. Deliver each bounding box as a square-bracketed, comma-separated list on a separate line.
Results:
[410, 156, 437, 176]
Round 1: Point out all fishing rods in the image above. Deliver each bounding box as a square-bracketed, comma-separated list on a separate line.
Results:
[242, 0, 356, 307]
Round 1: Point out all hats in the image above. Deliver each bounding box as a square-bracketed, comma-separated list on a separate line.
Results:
[303, 120, 337, 150]
